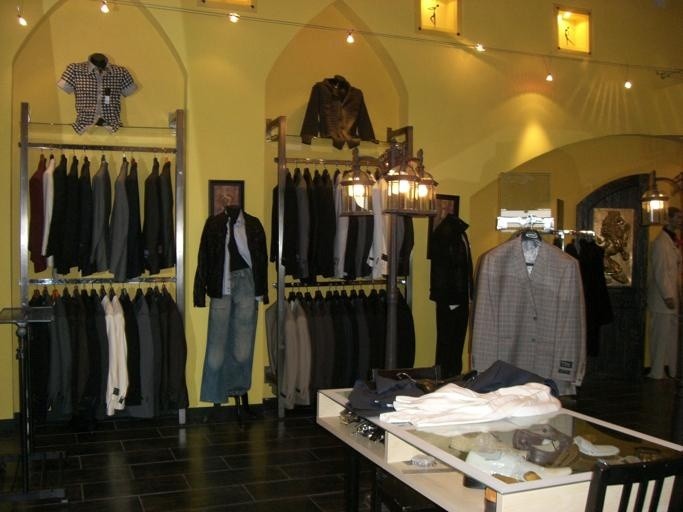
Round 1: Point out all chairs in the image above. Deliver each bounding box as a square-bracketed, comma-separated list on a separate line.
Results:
[370, 365, 441, 512]
[584, 455, 682, 512]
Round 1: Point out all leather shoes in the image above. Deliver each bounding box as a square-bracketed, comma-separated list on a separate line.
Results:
[448, 424, 642, 489]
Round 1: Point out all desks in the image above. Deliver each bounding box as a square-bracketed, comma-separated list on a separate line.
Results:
[315, 376, 683, 512]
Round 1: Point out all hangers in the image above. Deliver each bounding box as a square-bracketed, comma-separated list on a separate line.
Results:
[31, 279, 167, 303]
[295, 156, 369, 175]
[284, 279, 386, 304]
[38, 141, 170, 165]
[516, 227, 541, 266]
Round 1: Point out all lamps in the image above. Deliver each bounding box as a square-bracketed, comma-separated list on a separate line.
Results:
[640, 170, 683, 227]
[340, 138, 438, 216]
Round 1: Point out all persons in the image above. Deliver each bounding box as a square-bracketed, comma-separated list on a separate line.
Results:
[190, 203, 270, 419]
[648, 207, 682, 381]
[430, 213, 477, 376]
[55, 53, 137, 135]
